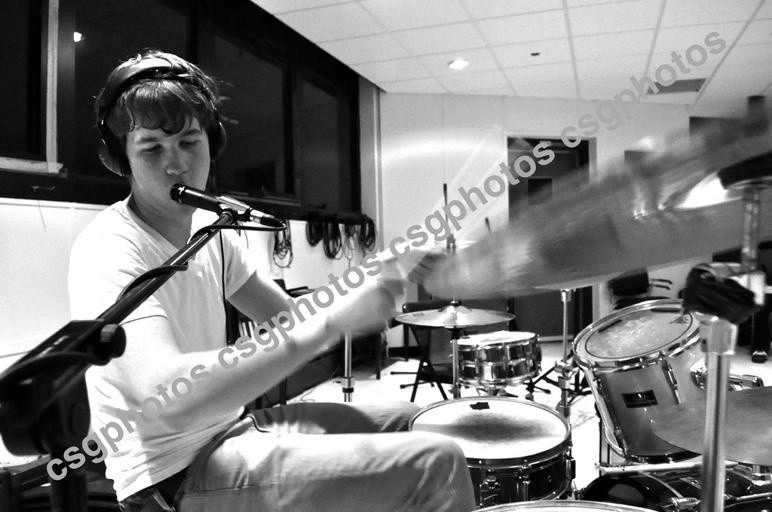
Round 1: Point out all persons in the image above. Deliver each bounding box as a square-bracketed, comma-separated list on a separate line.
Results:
[69, 51, 478, 512]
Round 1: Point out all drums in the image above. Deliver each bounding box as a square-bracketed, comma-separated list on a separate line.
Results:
[570, 296, 722, 467]
[406, 393, 577, 508]
[452, 328, 543, 386]
[575, 465, 770, 511]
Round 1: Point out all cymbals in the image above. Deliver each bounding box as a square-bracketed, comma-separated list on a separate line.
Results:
[395, 295, 513, 336]
[420, 111, 771, 304]
[647, 385, 772, 471]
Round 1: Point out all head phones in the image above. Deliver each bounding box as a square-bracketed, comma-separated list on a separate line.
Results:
[98, 58, 226, 176]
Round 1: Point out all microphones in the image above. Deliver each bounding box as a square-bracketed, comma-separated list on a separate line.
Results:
[170, 182, 285, 234]
